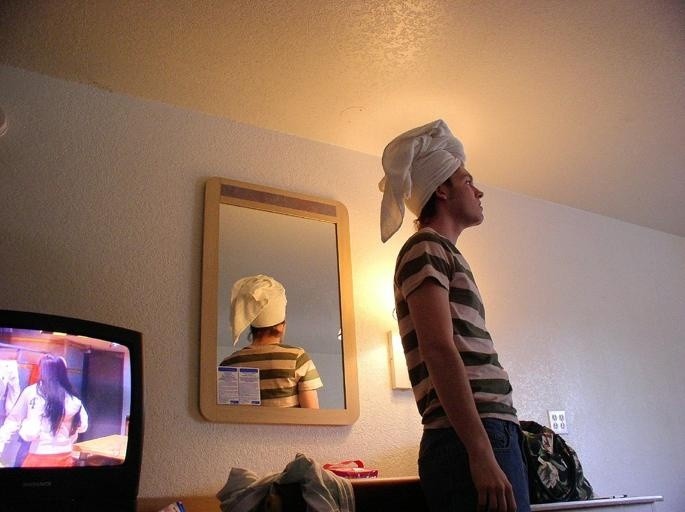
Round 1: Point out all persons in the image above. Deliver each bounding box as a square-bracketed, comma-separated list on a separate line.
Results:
[0, 352, 89, 468]
[218, 276, 324, 409]
[379, 119, 531, 512]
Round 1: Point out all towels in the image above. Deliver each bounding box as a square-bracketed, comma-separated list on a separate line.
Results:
[377, 119, 467, 245]
[228, 270, 288, 346]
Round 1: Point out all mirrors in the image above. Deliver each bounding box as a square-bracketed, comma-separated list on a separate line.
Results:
[198, 176, 360, 431]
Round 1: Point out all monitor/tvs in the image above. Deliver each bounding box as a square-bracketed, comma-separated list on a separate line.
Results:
[0, 308, 144, 512]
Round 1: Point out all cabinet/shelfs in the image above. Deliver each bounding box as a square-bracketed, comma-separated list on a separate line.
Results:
[531, 493, 664, 510]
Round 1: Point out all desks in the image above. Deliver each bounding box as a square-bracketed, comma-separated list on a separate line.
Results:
[280, 470, 425, 511]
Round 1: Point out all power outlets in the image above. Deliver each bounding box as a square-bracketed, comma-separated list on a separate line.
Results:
[548, 408, 569, 434]
